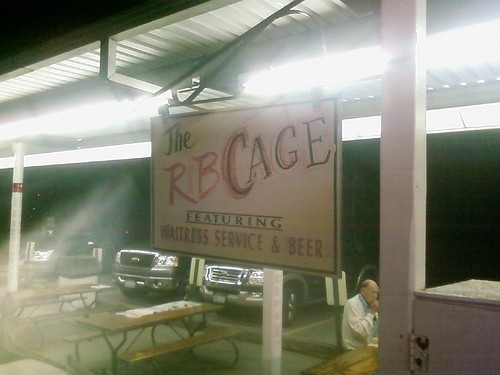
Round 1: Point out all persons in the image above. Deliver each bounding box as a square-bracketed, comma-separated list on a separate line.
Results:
[342, 279, 379, 350]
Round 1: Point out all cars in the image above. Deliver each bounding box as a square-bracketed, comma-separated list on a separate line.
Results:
[112, 238, 191, 303]
[199, 259, 340, 329]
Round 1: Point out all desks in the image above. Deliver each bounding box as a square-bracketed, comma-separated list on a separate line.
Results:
[6, 284, 114, 320]
[77, 300, 225, 375]
[300, 344, 378, 375]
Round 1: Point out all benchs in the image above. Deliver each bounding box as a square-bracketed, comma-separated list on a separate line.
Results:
[13, 297, 246, 375]
